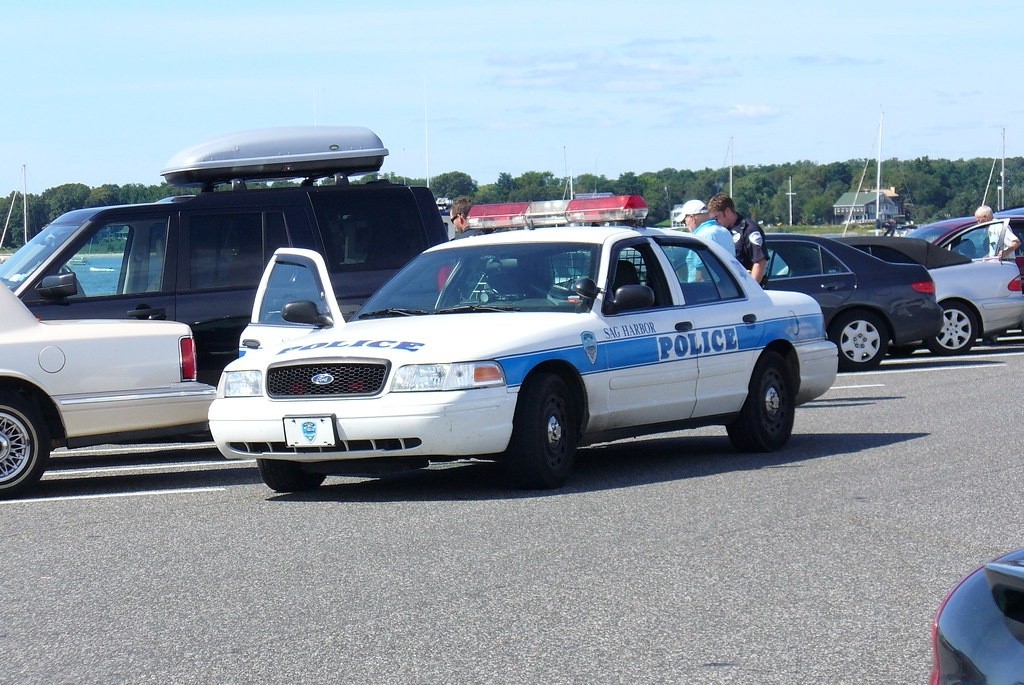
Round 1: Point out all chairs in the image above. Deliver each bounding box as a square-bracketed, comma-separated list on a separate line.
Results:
[959, 238, 977, 258]
[612, 261, 641, 296]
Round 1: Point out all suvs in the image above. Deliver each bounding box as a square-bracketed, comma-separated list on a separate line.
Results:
[0, 124, 454, 386]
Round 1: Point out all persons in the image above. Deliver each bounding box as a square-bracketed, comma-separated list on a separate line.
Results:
[707, 194, 770, 284]
[975, 205, 1020, 347]
[676, 199, 736, 284]
[450, 198, 500, 299]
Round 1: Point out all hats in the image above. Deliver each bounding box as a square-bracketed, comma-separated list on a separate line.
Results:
[675, 200, 708, 222]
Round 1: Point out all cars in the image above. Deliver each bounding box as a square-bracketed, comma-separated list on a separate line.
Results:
[905, 214, 1024, 275]
[930, 549, 1024, 685]
[669, 233, 944, 371]
[208, 194, 838, 490]
[0, 282, 217, 493]
[778, 235, 1024, 357]
[993, 205, 1024, 217]
